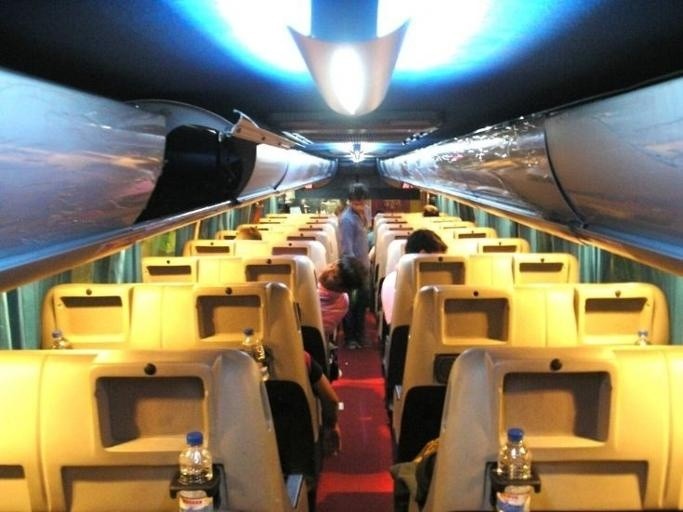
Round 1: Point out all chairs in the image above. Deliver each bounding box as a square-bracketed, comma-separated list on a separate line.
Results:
[40, 343, 311, 511]
[0, 343, 45, 511]
[660, 344, 683, 512]
[389, 343, 669, 512]
[37, 197, 338, 469]
[370, 208, 668, 446]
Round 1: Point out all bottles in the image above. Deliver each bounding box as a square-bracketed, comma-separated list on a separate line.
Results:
[497, 427, 531, 510]
[175, 433, 215, 512]
[634, 329, 651, 345]
[348, 286, 357, 305]
[239, 328, 269, 383]
[48, 329, 72, 348]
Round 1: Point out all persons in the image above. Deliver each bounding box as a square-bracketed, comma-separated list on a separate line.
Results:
[378, 228, 447, 329]
[335, 183, 371, 350]
[302, 351, 342, 458]
[315, 255, 368, 341]
[367, 209, 384, 264]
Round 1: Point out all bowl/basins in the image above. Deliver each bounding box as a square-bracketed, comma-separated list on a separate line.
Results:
[126, 98, 233, 137]
